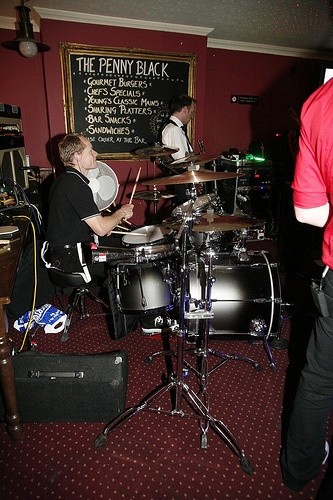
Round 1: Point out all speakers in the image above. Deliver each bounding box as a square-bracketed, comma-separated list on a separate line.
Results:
[12, 351, 130, 424]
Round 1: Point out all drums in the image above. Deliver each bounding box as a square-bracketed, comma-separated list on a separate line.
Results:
[121, 224, 175, 263]
[107, 250, 187, 315]
[171, 193, 227, 248]
[184, 245, 283, 343]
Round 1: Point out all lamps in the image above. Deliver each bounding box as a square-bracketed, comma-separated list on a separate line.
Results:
[0, 0, 51, 57]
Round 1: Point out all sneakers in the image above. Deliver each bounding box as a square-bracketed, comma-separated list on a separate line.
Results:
[142, 310, 180, 333]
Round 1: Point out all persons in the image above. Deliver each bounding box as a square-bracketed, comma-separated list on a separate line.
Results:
[162, 95, 196, 160]
[280, 78, 333, 500]
[46, 132, 176, 336]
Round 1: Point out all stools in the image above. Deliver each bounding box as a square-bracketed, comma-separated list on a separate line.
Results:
[46, 267, 112, 342]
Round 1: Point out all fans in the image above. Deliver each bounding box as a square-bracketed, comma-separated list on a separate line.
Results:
[85, 160, 120, 213]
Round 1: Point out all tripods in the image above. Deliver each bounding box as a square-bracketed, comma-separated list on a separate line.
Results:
[94, 183, 262, 476]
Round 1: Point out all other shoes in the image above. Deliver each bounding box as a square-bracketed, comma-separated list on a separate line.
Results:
[284, 440, 329, 491]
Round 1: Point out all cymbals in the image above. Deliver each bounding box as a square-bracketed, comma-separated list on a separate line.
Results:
[170, 153, 221, 167]
[131, 142, 180, 157]
[126, 188, 178, 201]
[168, 213, 266, 232]
[140, 170, 246, 185]
